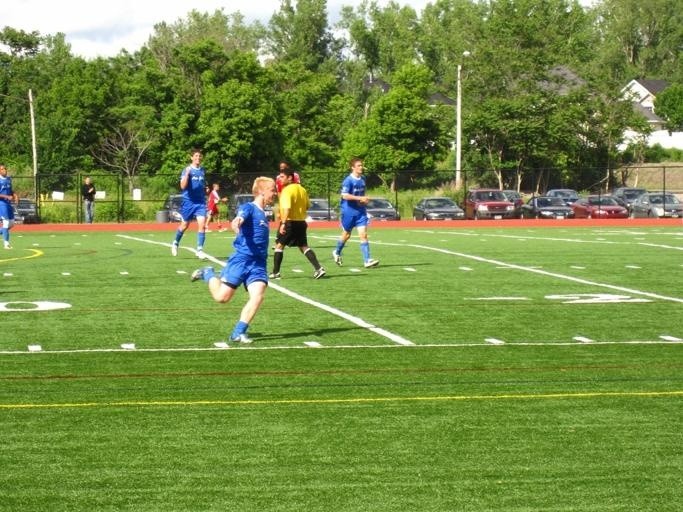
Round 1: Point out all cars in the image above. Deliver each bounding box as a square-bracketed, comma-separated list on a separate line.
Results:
[411, 196, 465, 221]
[364, 195, 401, 221]
[162, 192, 197, 223]
[230, 193, 275, 222]
[304, 198, 337, 221]
[461, 184, 682, 219]
[8, 197, 38, 225]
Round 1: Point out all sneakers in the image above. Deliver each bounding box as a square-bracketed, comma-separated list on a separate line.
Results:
[172, 242, 379, 344]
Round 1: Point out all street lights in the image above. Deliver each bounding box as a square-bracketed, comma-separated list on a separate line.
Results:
[453, 48, 471, 191]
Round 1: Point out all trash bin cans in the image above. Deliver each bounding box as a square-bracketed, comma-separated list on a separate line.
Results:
[156, 211, 170, 223]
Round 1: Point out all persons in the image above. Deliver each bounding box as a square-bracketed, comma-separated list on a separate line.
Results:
[172, 150, 210, 260]
[190, 176, 278, 344]
[82, 176, 96, 223]
[272, 160, 300, 249]
[206, 183, 228, 232]
[332, 158, 380, 268]
[269, 168, 325, 280]
[0, 163, 20, 250]
